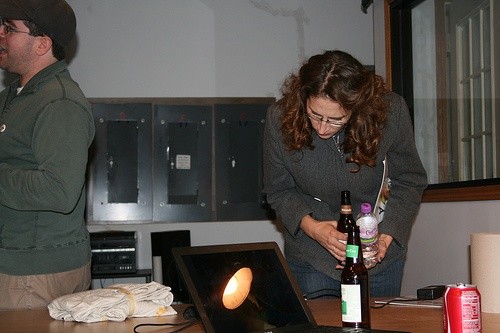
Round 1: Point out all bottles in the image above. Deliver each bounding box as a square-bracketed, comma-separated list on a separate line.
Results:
[336, 190, 355, 266]
[355, 203, 379, 270]
[341, 225, 371, 333]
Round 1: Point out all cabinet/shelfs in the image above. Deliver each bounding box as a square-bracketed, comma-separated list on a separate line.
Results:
[83, 97, 276, 224]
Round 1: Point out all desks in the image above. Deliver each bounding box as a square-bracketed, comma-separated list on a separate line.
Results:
[0, 297, 500, 333]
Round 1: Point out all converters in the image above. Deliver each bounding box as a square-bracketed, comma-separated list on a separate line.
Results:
[416, 284, 445, 300]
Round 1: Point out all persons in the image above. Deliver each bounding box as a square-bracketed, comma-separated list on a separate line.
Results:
[262, 50, 428, 299]
[0, 0, 97, 311]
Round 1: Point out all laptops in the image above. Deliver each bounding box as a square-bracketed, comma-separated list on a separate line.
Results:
[167, 240, 412, 333]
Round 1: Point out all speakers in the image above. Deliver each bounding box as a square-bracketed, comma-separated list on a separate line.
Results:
[150, 229, 191, 304]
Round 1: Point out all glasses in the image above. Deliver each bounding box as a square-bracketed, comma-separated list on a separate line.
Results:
[0, 20, 44, 36]
[306, 113, 346, 127]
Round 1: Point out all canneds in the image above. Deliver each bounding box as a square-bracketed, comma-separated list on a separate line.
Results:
[442, 282, 482, 333]
